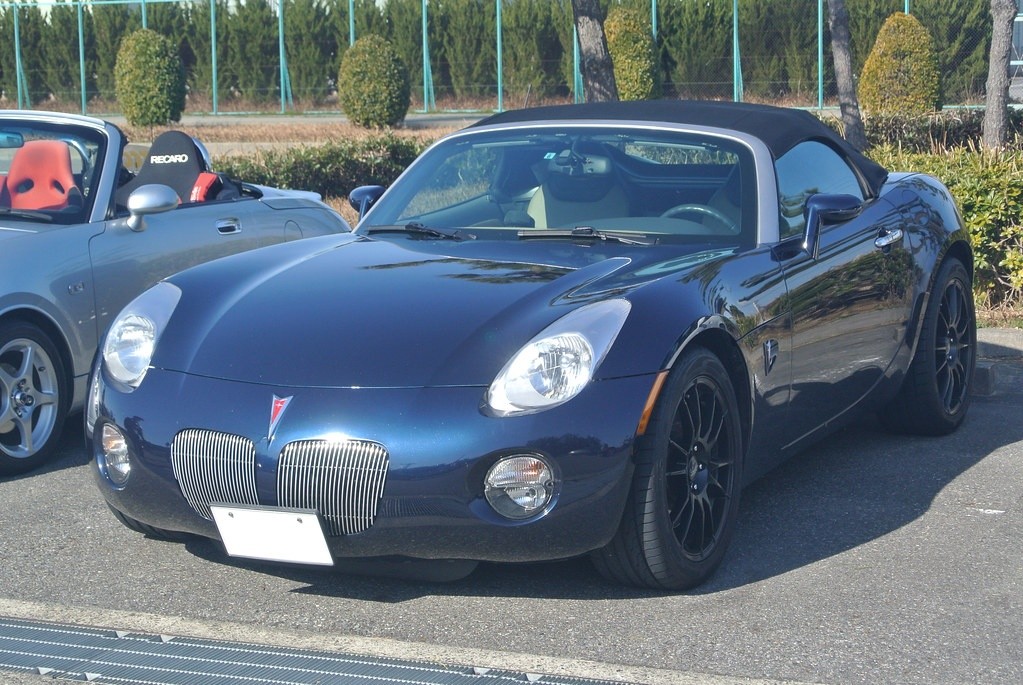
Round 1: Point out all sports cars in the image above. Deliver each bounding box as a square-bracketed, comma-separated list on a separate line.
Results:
[0, 108, 352, 478]
[83, 100, 978, 593]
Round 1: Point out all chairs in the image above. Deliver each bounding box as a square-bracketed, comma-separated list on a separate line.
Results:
[527, 177, 630, 229]
[115, 131, 218, 207]
[0, 140, 76, 208]
